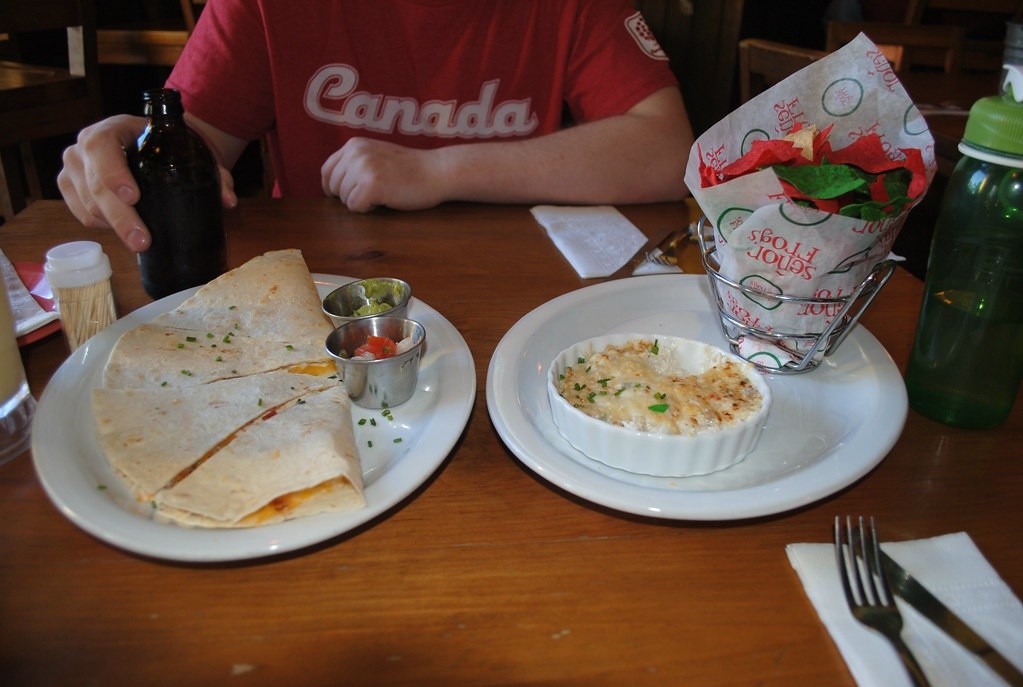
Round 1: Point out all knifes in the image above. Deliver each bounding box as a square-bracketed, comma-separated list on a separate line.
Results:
[841, 527, 1023, 687]
[636, 231, 678, 268]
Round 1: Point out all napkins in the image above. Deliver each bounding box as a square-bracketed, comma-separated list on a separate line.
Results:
[530, 205, 649, 279]
[784, 531, 1023, 687]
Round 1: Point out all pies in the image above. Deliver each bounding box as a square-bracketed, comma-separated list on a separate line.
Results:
[91, 248, 367, 528]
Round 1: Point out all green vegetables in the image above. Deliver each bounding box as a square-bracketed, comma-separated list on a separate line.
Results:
[756, 154, 920, 223]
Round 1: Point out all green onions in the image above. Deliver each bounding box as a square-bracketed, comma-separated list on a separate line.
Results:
[558, 337, 669, 414]
[158, 305, 403, 450]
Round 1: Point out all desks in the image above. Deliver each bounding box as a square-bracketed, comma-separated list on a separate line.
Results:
[0, 59, 102, 144]
[899, 73, 1000, 162]
[96, 30, 188, 65]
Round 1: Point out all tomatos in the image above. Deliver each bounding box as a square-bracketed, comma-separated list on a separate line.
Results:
[353, 335, 395, 359]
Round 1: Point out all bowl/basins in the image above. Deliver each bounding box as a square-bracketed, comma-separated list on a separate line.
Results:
[547, 334, 772, 477]
[322, 277, 412, 328]
[325, 315, 426, 410]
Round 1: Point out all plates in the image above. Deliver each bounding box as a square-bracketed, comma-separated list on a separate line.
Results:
[13, 261, 61, 346]
[486, 274, 910, 521]
[31, 273, 477, 561]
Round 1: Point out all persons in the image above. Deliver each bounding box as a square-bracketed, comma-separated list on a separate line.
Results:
[58, 0, 693, 253]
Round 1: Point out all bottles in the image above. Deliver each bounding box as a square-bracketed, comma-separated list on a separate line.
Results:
[128, 88, 225, 301]
[905, 63, 1023, 428]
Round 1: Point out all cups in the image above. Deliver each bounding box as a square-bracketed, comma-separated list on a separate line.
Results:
[0, 266, 39, 464]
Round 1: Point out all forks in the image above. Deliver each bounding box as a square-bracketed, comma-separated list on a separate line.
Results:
[835, 515, 929, 687]
[645, 229, 695, 266]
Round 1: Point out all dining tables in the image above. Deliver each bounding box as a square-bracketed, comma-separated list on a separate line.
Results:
[0, 197, 1023, 687]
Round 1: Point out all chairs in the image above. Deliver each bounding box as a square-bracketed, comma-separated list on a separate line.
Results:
[739, 20, 961, 108]
